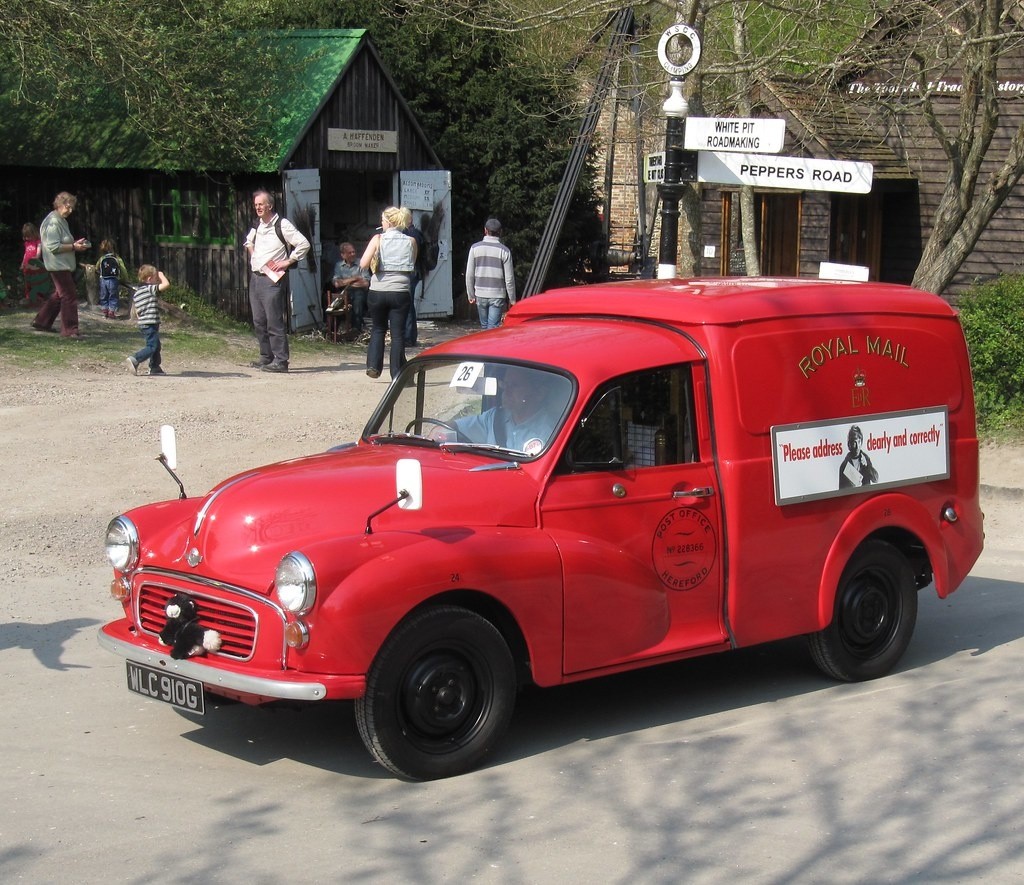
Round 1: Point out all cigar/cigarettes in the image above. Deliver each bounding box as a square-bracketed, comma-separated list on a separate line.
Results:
[376, 226, 383, 230]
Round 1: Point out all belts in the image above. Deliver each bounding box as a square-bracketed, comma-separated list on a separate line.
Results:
[255, 272, 267, 276]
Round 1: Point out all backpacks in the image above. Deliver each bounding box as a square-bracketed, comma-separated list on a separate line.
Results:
[410, 228, 439, 271]
[100, 253, 120, 278]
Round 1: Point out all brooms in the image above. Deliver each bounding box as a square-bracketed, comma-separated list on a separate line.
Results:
[291, 204, 324, 332]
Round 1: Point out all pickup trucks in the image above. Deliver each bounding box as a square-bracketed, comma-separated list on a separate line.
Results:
[92, 273, 987, 786]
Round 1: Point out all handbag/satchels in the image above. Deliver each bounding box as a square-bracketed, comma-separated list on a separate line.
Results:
[370, 232, 382, 274]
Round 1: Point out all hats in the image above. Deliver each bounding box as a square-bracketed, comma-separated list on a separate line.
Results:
[485, 219, 501, 232]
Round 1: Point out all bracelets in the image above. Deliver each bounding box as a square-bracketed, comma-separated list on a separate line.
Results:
[72, 243, 75, 251]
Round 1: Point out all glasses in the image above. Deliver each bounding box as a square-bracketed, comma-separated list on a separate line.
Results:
[499, 381, 525, 390]
[62, 203, 75, 211]
[252, 202, 269, 208]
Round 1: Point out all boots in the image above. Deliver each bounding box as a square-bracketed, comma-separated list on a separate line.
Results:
[103, 309, 109, 317]
[109, 311, 116, 318]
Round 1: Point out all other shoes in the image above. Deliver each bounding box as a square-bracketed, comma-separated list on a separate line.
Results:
[68, 332, 85, 340]
[261, 362, 288, 372]
[150, 368, 166, 375]
[127, 356, 139, 376]
[366, 368, 379, 379]
[250, 358, 270, 369]
[31, 318, 58, 332]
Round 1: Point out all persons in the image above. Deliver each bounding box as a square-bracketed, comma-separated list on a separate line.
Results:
[359, 206, 416, 388]
[243, 192, 310, 372]
[21, 222, 42, 304]
[431, 369, 555, 451]
[322, 242, 370, 335]
[127, 265, 170, 376]
[838, 426, 879, 488]
[31, 192, 91, 341]
[96, 239, 128, 319]
[466, 219, 516, 330]
[402, 214, 426, 347]
